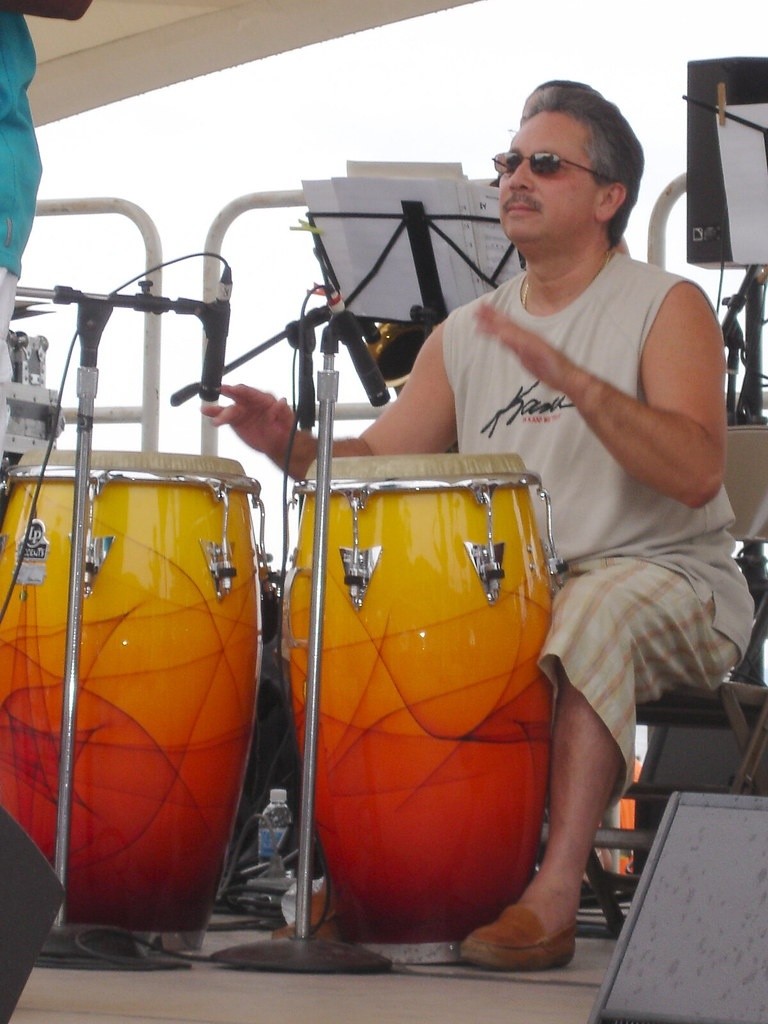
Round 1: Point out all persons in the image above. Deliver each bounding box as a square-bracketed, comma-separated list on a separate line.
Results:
[202, 79, 757, 970]
[0, 0, 99, 522]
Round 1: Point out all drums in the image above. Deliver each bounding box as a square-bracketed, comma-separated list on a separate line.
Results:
[1, 446, 265, 957]
[282, 450, 558, 967]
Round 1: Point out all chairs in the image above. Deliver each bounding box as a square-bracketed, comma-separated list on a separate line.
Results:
[534, 423, 768, 939]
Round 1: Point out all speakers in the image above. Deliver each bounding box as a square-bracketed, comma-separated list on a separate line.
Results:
[687, 56, 768, 270]
[588, 790, 768, 1024]
[0, 806, 67, 1024]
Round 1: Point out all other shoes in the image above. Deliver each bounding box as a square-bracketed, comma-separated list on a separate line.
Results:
[270, 887, 343, 943]
[458, 905, 577, 970]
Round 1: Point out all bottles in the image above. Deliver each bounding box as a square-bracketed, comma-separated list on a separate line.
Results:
[259, 788, 293, 903]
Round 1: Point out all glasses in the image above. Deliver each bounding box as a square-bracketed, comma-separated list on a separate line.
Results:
[492, 152, 616, 185]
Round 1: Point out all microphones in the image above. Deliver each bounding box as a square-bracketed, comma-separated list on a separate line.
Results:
[200, 267, 233, 402]
[322, 284, 390, 408]
[359, 319, 381, 343]
